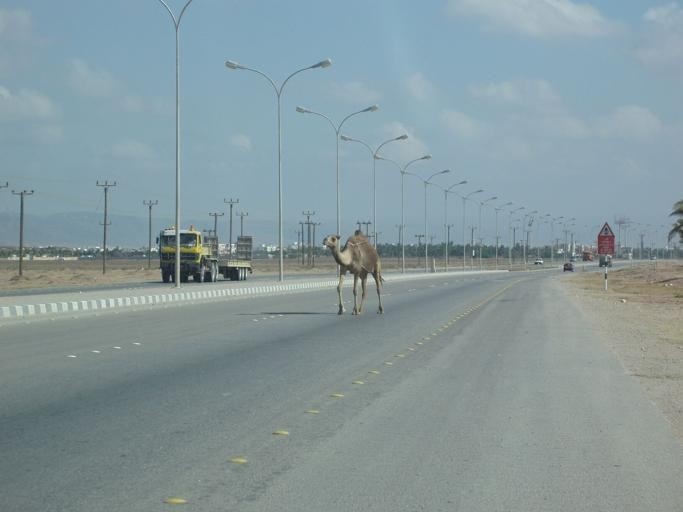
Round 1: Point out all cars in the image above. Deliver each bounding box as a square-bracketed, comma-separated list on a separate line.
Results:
[565, 257, 577, 271]
[649, 255, 655, 260]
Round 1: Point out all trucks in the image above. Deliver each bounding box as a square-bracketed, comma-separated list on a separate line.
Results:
[583, 251, 593, 261]
[155, 226, 252, 283]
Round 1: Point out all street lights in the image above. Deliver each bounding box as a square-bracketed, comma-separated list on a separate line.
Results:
[292, 105, 377, 275]
[372, 155, 431, 270]
[225, 57, 333, 284]
[338, 131, 408, 251]
[445, 179, 566, 270]
[411, 167, 448, 271]
